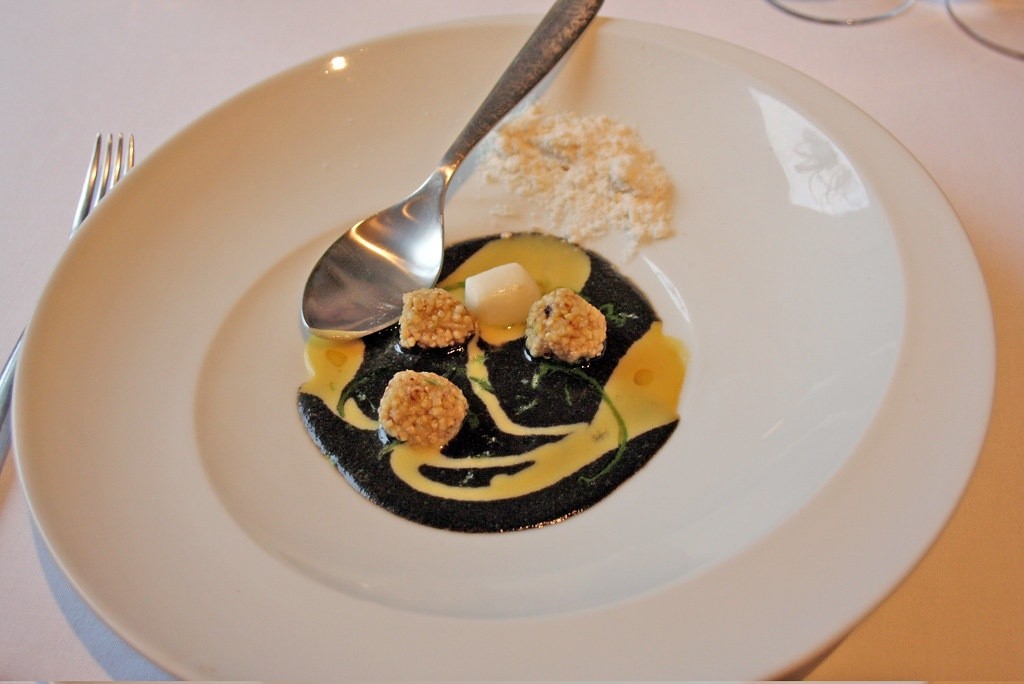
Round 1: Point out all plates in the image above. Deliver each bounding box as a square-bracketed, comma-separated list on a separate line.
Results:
[13, 17, 997, 683]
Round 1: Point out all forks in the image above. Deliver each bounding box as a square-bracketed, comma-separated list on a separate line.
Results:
[2, 134, 137, 426]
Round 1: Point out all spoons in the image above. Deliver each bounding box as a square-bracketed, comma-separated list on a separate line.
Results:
[302, 0, 603, 341]
